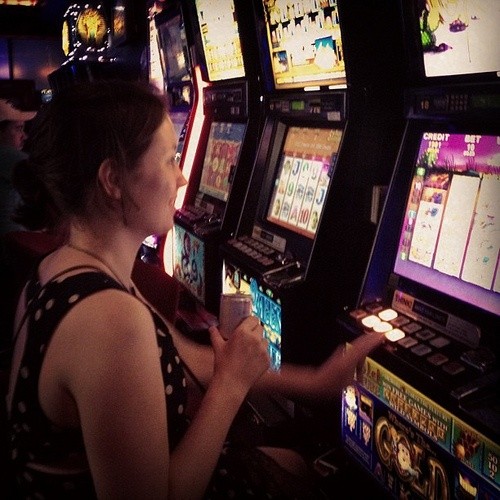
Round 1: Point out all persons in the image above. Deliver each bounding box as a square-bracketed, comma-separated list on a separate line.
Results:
[0, 78, 387, 500]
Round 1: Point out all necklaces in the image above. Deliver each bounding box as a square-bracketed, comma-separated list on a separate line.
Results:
[66, 242, 127, 288]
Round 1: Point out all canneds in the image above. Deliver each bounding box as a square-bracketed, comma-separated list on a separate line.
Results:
[218, 293, 253, 340]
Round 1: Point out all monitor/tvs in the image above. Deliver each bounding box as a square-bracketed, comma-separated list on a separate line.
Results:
[151, 0, 499, 314]
